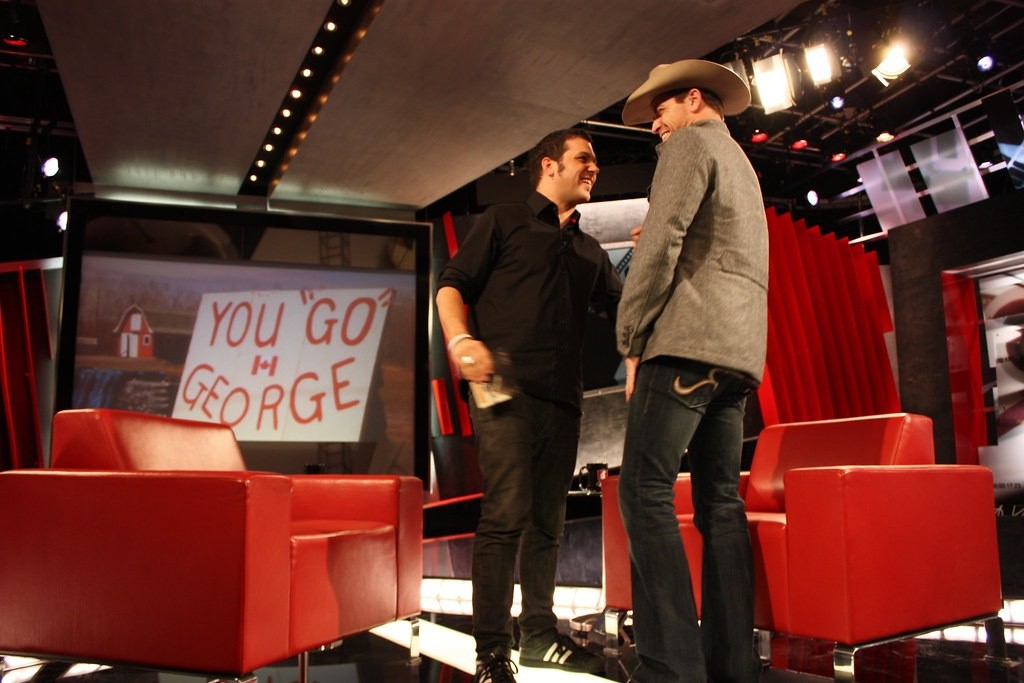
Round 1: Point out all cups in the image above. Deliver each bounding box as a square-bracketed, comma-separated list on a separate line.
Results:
[578, 463, 608, 492]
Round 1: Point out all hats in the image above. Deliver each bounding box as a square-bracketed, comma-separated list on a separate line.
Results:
[622, 59, 751, 126]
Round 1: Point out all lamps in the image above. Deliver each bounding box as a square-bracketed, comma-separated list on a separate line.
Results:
[723, 13, 914, 116]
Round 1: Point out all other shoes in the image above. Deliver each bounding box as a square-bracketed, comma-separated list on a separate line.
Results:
[602, 652, 648, 683]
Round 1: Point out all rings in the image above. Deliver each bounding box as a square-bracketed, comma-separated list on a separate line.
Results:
[461, 357, 475, 367]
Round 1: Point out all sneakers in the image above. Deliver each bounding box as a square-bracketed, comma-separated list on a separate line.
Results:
[473, 655, 518, 683]
[519, 632, 607, 674]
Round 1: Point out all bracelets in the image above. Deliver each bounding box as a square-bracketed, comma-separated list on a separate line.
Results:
[446, 333, 475, 351]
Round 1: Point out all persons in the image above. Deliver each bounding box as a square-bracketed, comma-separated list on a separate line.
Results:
[614, 58, 770, 683]
[432, 129, 642, 683]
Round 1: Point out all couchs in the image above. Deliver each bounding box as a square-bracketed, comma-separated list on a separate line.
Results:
[601, 412, 1005, 683]
[0, 408, 425, 683]
